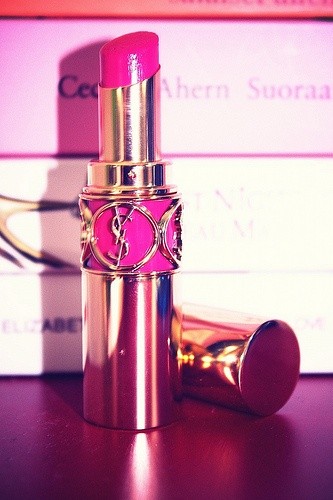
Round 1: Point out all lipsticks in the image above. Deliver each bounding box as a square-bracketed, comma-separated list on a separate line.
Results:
[76, 31, 299, 431]
